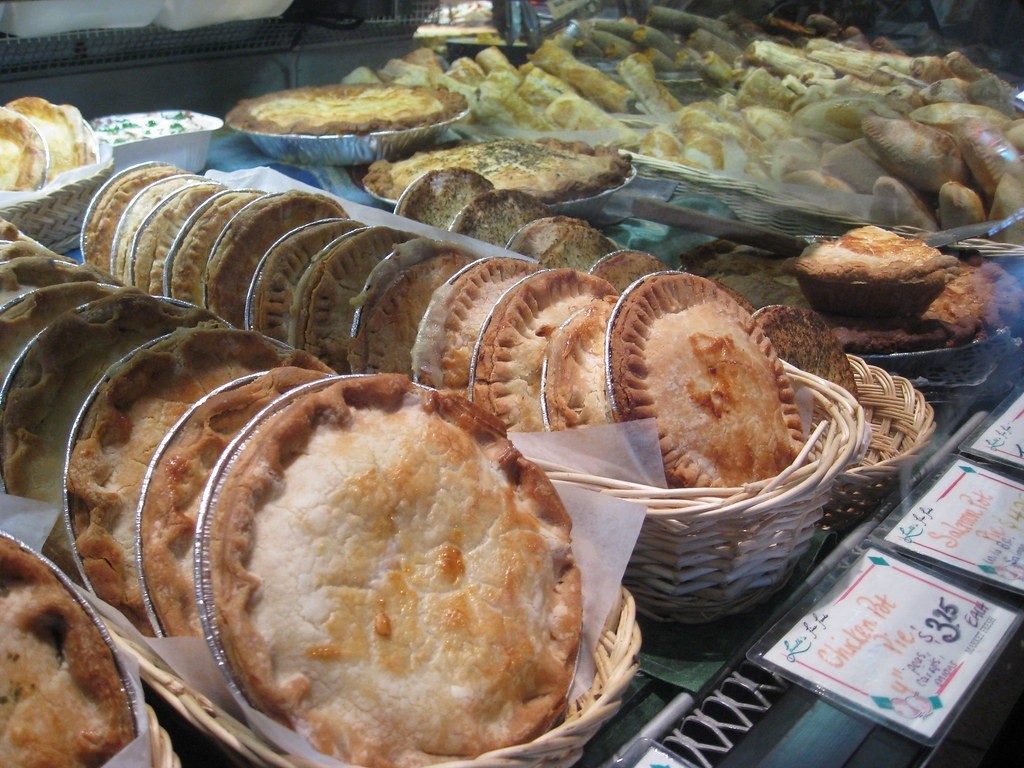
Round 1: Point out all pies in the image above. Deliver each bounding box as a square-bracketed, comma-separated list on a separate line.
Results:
[346, 5, 988, 132]
[0, 82, 1024, 768]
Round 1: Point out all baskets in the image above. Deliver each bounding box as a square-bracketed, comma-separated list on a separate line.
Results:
[613, 146, 1024, 285]
[810, 353, 938, 537]
[0, 209, 642, 768]
[0, 156, 116, 255]
[520, 356, 873, 626]
